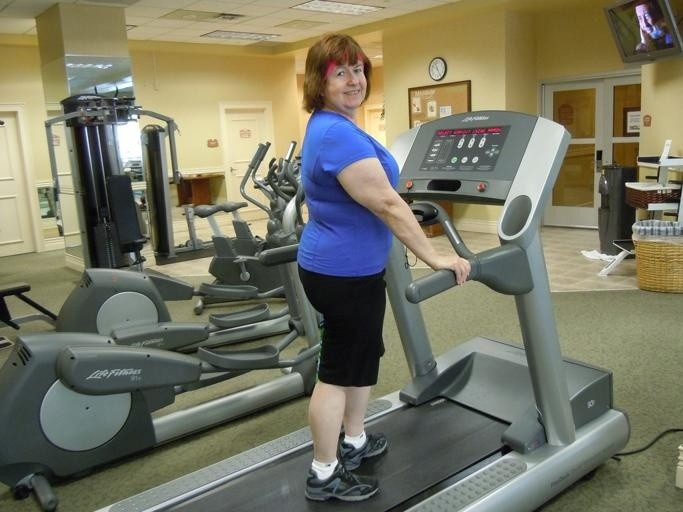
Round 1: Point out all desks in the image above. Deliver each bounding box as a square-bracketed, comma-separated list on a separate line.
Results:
[177, 175, 210, 207]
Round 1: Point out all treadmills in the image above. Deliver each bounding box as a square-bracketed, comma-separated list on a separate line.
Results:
[94, 109, 631, 512]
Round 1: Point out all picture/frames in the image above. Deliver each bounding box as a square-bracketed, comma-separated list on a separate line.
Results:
[623, 107, 642, 136]
[408, 79, 471, 129]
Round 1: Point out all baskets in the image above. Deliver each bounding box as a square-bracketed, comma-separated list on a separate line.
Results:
[624, 181, 680, 210]
[630, 221, 682, 293]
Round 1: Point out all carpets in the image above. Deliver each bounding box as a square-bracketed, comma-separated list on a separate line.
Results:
[1, 242, 683, 511]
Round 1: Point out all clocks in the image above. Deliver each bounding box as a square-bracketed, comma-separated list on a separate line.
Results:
[428, 57, 447, 80]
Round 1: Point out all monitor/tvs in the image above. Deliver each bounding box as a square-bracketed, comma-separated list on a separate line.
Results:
[603, 0, 683, 64]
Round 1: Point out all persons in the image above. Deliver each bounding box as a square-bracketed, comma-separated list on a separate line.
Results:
[632, 1, 675, 53]
[294, 28, 471, 503]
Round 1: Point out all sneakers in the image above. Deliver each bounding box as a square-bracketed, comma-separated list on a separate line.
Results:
[305, 461, 380, 502]
[338, 432, 387, 471]
